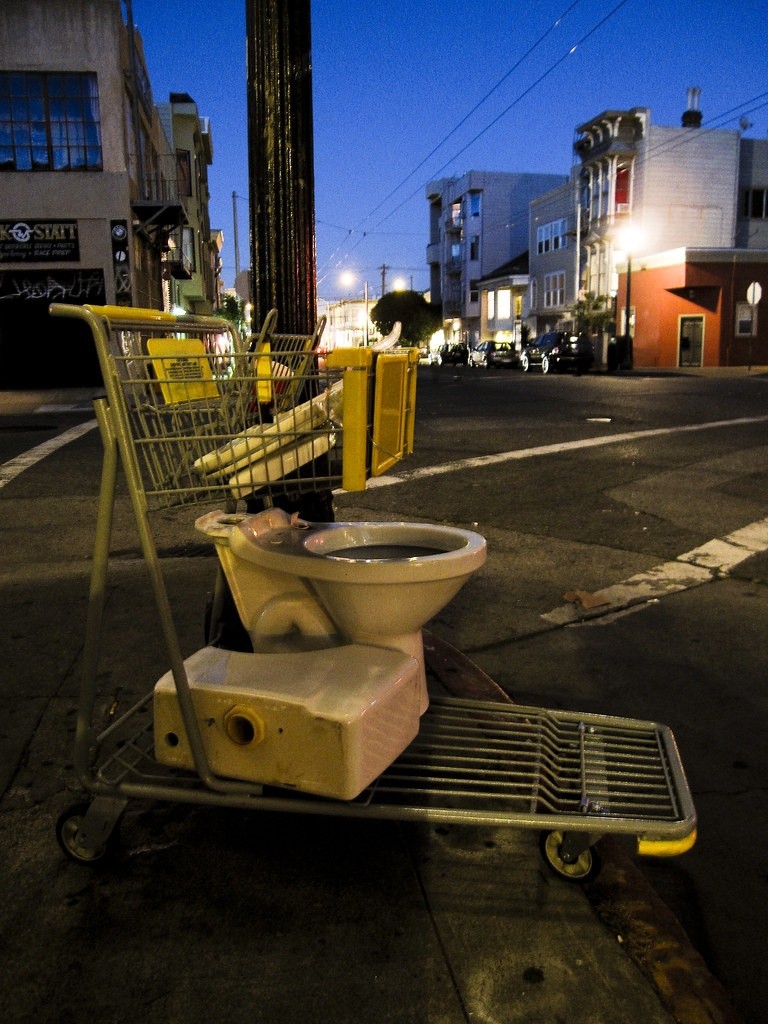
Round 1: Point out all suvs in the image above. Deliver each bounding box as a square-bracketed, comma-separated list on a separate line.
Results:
[520, 331, 595, 374]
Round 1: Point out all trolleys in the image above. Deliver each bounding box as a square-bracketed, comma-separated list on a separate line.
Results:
[47, 300, 702, 883]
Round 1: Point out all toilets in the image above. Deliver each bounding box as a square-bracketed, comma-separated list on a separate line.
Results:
[152, 508, 489, 802]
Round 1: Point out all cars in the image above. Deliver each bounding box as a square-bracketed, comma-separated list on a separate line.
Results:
[469, 341, 518, 370]
[428, 343, 469, 366]
